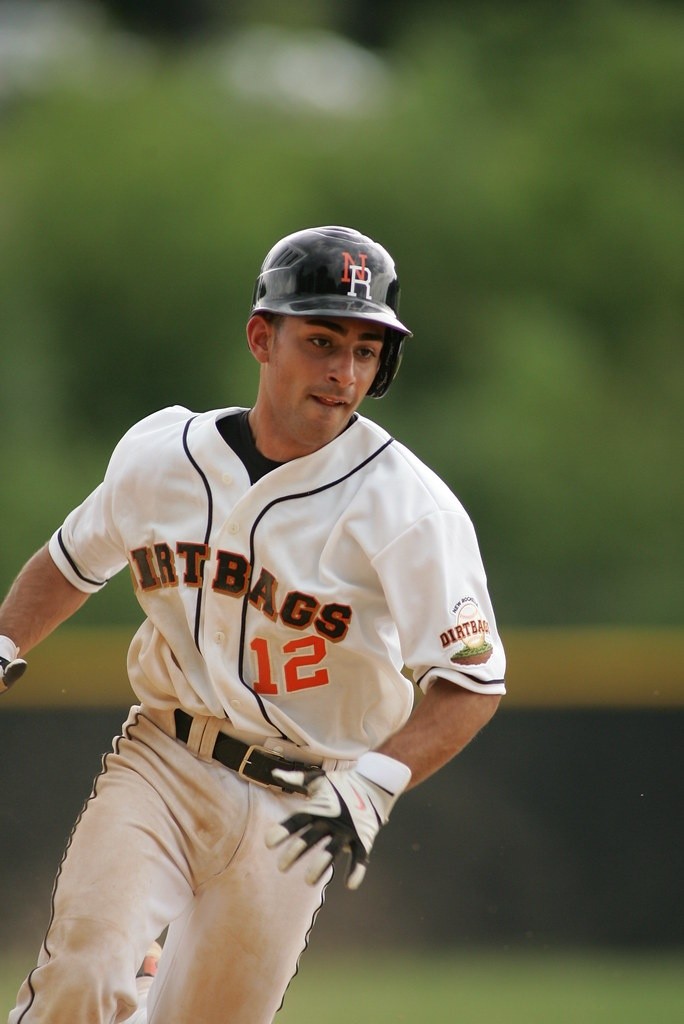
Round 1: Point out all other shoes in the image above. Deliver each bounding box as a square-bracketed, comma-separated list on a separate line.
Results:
[135, 939, 162, 981]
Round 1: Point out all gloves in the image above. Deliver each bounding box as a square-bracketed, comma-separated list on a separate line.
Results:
[0, 635, 28, 696]
[265, 751, 412, 890]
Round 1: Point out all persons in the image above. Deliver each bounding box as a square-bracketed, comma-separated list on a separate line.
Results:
[1, 223, 509, 1023]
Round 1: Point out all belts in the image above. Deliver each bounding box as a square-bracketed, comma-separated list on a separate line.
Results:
[174, 708, 321, 796]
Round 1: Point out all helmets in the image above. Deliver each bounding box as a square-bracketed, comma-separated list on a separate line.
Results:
[249, 225, 414, 399]
